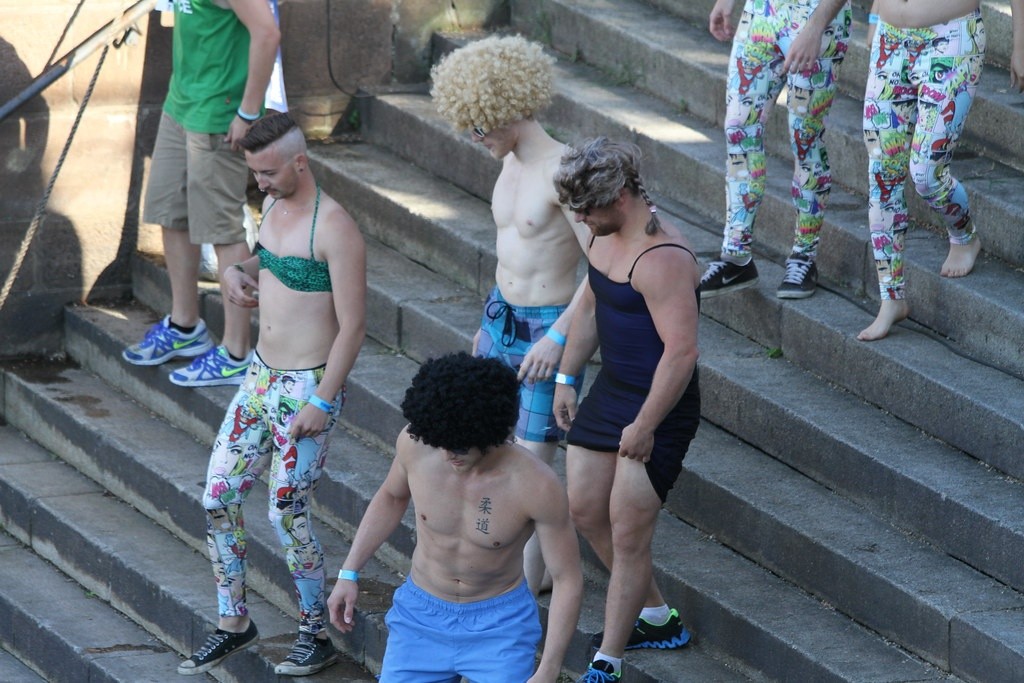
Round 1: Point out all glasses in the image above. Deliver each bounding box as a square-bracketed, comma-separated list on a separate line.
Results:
[450, 447, 470, 455]
[569, 206, 591, 216]
[472, 127, 486, 138]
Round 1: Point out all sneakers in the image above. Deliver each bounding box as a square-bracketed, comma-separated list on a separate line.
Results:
[575, 658, 621, 683]
[121, 314, 215, 365]
[591, 609, 691, 651]
[699, 255, 758, 298]
[178, 618, 260, 676]
[776, 254, 819, 298]
[274, 632, 337, 676]
[168, 345, 254, 386]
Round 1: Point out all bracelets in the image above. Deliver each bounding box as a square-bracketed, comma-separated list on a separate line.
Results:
[236, 106, 262, 123]
[555, 372, 576, 386]
[337, 568, 359, 582]
[546, 327, 566, 347]
[308, 394, 334, 415]
[868, 12, 878, 24]
[233, 263, 248, 290]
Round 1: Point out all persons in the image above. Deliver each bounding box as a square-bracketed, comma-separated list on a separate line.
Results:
[175, 111, 368, 675]
[428, 33, 594, 606]
[552, 134, 703, 683]
[699, 0, 853, 298]
[327, 352, 583, 682]
[123, 0, 284, 387]
[857, 0, 1024, 342]
